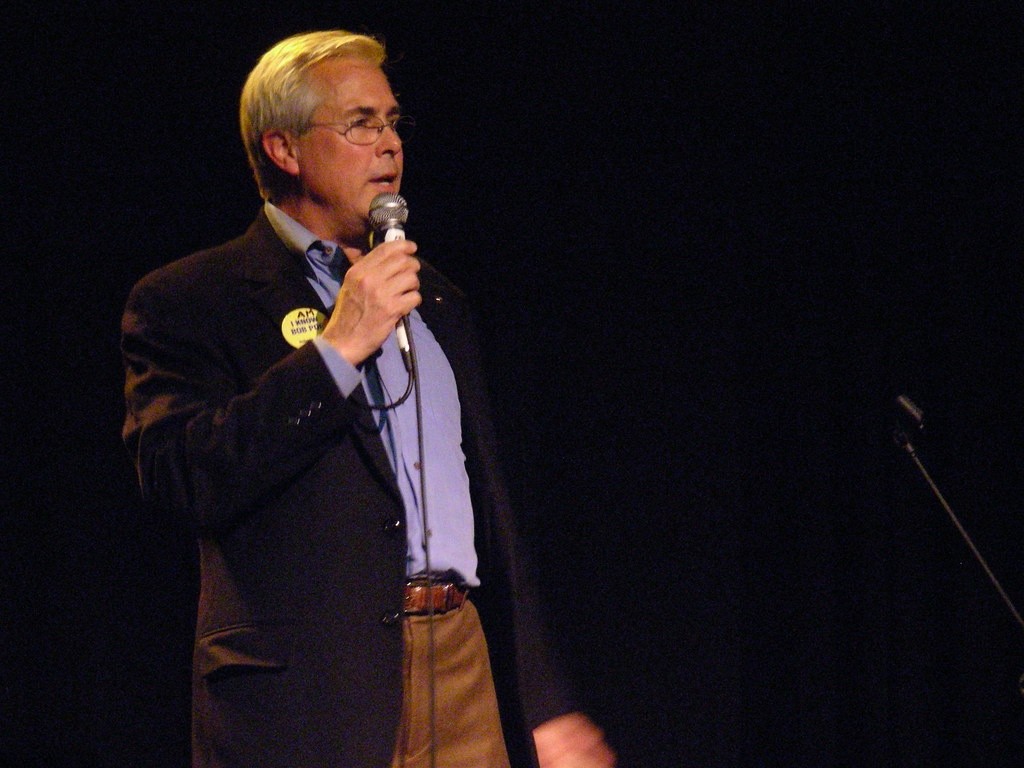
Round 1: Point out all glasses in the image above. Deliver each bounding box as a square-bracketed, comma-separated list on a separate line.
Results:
[298, 115, 418, 144]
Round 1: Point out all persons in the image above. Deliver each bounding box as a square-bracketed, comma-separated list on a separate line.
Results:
[121, 30, 619, 768]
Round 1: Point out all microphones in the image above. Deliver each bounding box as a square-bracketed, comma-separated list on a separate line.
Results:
[369, 191, 418, 373]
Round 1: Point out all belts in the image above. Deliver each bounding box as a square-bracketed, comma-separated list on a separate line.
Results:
[405, 578, 470, 615]
